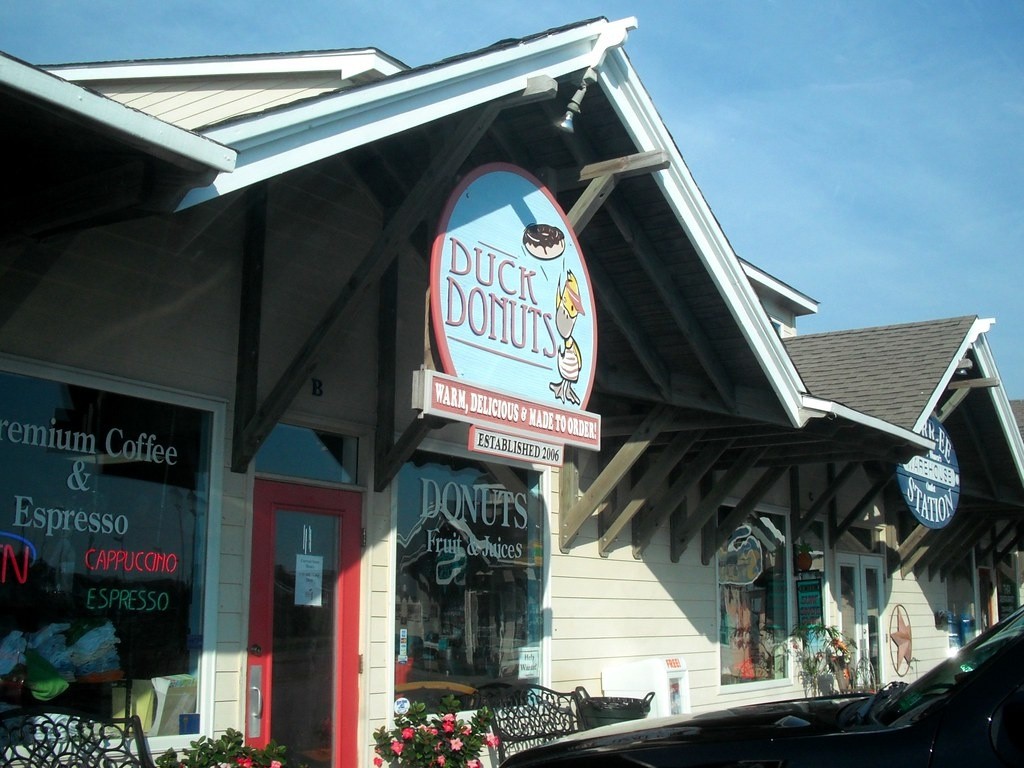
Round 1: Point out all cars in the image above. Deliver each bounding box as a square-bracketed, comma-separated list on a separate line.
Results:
[422, 630, 449, 660]
[501, 604, 1023, 768]
[436, 637, 488, 677]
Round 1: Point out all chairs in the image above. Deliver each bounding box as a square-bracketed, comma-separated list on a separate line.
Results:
[488, 682, 591, 766]
[0, 708, 155, 768]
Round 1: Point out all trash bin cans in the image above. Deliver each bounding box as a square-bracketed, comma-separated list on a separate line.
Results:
[575, 685, 656, 732]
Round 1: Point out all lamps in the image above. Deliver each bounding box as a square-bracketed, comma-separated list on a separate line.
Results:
[552, 88, 586, 134]
[955, 352, 968, 376]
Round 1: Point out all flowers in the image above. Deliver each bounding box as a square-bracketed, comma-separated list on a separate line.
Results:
[155, 728, 288, 768]
[369, 692, 499, 767]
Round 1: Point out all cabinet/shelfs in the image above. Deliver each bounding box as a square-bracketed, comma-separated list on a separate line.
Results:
[1, 670, 128, 735]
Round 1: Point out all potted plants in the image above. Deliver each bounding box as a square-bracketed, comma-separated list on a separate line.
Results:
[825, 625, 858, 695]
